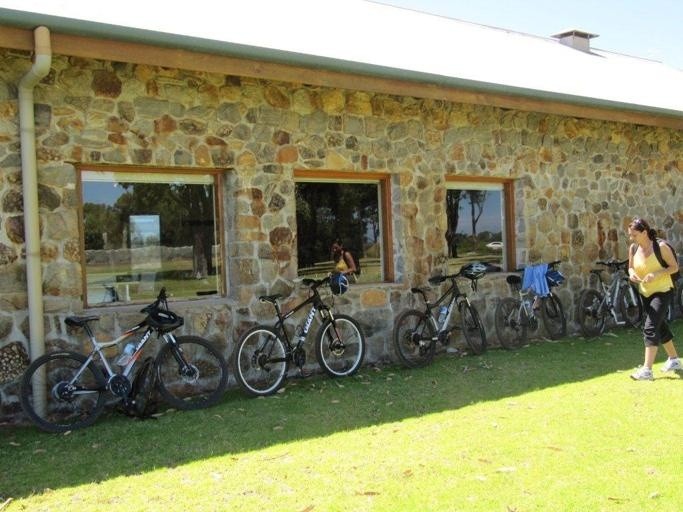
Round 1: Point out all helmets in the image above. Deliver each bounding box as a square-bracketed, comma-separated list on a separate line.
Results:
[146, 307, 185, 333]
[545, 270, 564, 287]
[462, 263, 487, 281]
[327, 270, 350, 297]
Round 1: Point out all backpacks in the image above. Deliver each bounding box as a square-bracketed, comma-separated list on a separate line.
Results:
[343, 248, 361, 277]
[632, 237, 680, 282]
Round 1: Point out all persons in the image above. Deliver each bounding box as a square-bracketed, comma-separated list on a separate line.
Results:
[326, 239, 357, 283]
[623, 217, 682, 381]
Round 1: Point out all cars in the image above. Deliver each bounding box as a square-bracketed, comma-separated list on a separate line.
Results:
[484, 241, 503, 251]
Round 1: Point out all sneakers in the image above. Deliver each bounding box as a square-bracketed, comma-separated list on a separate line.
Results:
[630, 367, 652, 381]
[660, 357, 680, 373]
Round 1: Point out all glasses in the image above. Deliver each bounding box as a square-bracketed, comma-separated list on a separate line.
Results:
[632, 216, 641, 225]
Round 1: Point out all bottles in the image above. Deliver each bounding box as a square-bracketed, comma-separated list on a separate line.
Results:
[117, 338, 137, 367]
[438, 303, 448, 324]
[525, 297, 531, 314]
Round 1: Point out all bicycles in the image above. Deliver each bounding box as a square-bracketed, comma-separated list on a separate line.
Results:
[666, 270, 682, 324]
[102, 283, 118, 300]
[574, 259, 643, 339]
[232, 273, 366, 398]
[493, 259, 569, 352]
[16, 287, 230, 435]
[391, 267, 489, 371]
